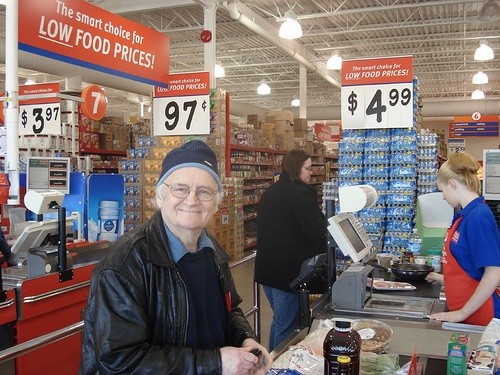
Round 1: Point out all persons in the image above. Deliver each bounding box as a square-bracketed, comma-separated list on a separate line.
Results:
[255, 149, 334, 352]
[77, 139, 273, 375]
[426, 152, 500, 326]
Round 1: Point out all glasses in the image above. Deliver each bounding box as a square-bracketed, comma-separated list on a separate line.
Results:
[302, 166, 312, 171]
[163, 181, 217, 202]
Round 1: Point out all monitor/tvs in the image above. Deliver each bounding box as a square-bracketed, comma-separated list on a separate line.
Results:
[11, 219, 74, 255]
[339, 219, 366, 253]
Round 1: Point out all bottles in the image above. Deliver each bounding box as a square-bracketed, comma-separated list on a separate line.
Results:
[231, 149, 321, 207]
[323, 321, 363, 375]
[321, 126, 442, 276]
[96, 199, 120, 243]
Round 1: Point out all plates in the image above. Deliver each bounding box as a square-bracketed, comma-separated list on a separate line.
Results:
[373, 280, 416, 292]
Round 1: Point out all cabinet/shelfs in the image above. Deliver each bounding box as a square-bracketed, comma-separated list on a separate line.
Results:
[79, 146, 127, 174]
[230, 143, 337, 250]
[1, 92, 84, 171]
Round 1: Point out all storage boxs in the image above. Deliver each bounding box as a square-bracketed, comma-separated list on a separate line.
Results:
[81, 116, 332, 261]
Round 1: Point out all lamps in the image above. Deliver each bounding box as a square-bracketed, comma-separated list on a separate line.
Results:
[478, 0, 500, 20]
[257, 79, 271, 93]
[471, 71, 488, 84]
[471, 89, 484, 99]
[473, 40, 495, 61]
[291, 98, 300, 106]
[327, 53, 343, 69]
[278, 11, 303, 39]
[215, 64, 225, 78]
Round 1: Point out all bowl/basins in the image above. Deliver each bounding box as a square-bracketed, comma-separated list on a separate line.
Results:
[390, 263, 434, 280]
[350, 318, 393, 354]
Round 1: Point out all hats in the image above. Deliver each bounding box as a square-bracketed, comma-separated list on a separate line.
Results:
[156, 139, 222, 192]
